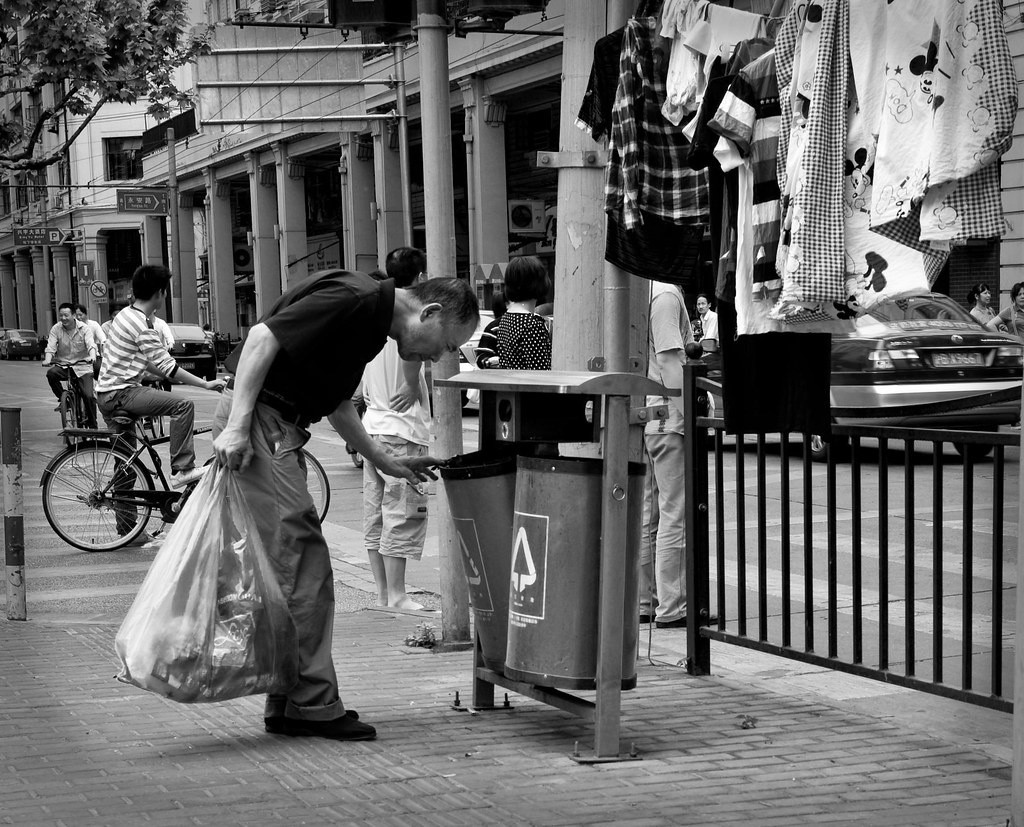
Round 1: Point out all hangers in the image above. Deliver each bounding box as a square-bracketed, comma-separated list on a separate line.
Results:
[749, 17, 784, 60]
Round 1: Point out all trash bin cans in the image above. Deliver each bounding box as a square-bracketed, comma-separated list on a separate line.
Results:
[504, 452, 646, 691]
[439, 441, 560, 672]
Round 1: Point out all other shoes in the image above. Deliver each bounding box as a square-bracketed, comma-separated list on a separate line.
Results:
[119, 527, 160, 547]
[656, 613, 718, 629]
[286, 717, 377, 740]
[169, 466, 213, 491]
[54, 401, 70, 412]
[639, 614, 657, 624]
[265, 705, 359, 732]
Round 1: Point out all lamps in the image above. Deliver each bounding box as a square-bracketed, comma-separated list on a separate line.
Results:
[47, 122, 57, 134]
[273, 224, 283, 240]
[370, 201, 381, 220]
[11, 271, 55, 287]
[247, 232, 255, 246]
[73, 266, 77, 277]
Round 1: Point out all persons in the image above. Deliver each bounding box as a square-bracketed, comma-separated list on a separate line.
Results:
[986, 281, 1024, 430]
[100, 311, 123, 337]
[140, 308, 176, 430]
[73, 304, 107, 381]
[476, 291, 508, 368]
[94, 263, 227, 547]
[346, 269, 389, 458]
[351, 248, 443, 618]
[498, 255, 551, 370]
[639, 281, 718, 628]
[696, 292, 718, 418]
[967, 282, 1008, 333]
[211, 267, 480, 741]
[41, 303, 100, 429]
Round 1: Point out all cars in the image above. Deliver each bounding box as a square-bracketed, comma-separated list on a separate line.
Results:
[0, 327, 42, 361]
[704, 290, 1024, 462]
[167, 323, 217, 382]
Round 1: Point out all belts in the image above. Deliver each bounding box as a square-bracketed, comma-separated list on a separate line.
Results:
[225, 379, 310, 432]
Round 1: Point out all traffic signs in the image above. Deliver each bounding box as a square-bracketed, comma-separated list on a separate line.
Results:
[116, 189, 169, 214]
[13, 227, 68, 247]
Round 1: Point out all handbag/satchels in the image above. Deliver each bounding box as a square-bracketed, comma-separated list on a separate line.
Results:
[115, 459, 296, 703]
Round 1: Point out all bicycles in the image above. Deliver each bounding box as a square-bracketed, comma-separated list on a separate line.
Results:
[42, 359, 93, 447]
[39, 376, 332, 553]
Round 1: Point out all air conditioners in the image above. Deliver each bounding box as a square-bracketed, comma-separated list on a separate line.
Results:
[508, 199, 547, 233]
[233, 242, 255, 272]
